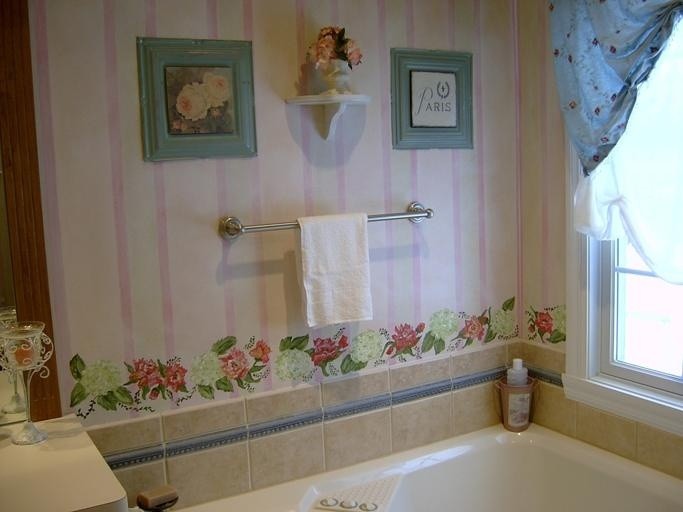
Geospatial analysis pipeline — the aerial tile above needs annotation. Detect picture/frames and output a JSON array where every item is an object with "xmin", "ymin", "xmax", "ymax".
[
  {"xmin": 388, "ymin": 44, "xmax": 474, "ymax": 150},
  {"xmin": 134, "ymin": 33, "xmax": 256, "ymax": 162}
]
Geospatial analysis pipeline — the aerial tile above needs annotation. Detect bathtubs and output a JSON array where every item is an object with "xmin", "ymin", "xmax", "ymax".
[{"xmin": 295, "ymin": 418, "xmax": 683, "ymax": 512}]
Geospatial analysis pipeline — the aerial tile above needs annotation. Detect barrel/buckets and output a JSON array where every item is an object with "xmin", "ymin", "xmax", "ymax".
[{"xmin": 494, "ymin": 378, "xmax": 539, "ymax": 431}]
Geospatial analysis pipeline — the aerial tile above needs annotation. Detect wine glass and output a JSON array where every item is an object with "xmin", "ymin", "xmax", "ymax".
[
  {"xmin": 1, "ymin": 320, "xmax": 55, "ymax": 446},
  {"xmin": 0, "ymin": 306, "xmax": 28, "ymax": 414}
]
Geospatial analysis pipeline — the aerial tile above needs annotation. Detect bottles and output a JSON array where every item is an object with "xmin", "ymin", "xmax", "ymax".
[{"xmin": 506, "ymin": 358, "xmax": 528, "ymax": 387}]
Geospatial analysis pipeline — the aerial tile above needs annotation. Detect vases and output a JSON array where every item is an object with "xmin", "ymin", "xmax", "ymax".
[{"xmin": 315, "ymin": 60, "xmax": 352, "ymax": 94}]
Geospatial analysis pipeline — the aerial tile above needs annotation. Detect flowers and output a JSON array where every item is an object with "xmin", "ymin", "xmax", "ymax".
[{"xmin": 306, "ymin": 23, "xmax": 363, "ymax": 69}]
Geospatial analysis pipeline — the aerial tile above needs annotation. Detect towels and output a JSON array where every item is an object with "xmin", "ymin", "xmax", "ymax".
[{"xmin": 295, "ymin": 212, "xmax": 373, "ymax": 329}]
